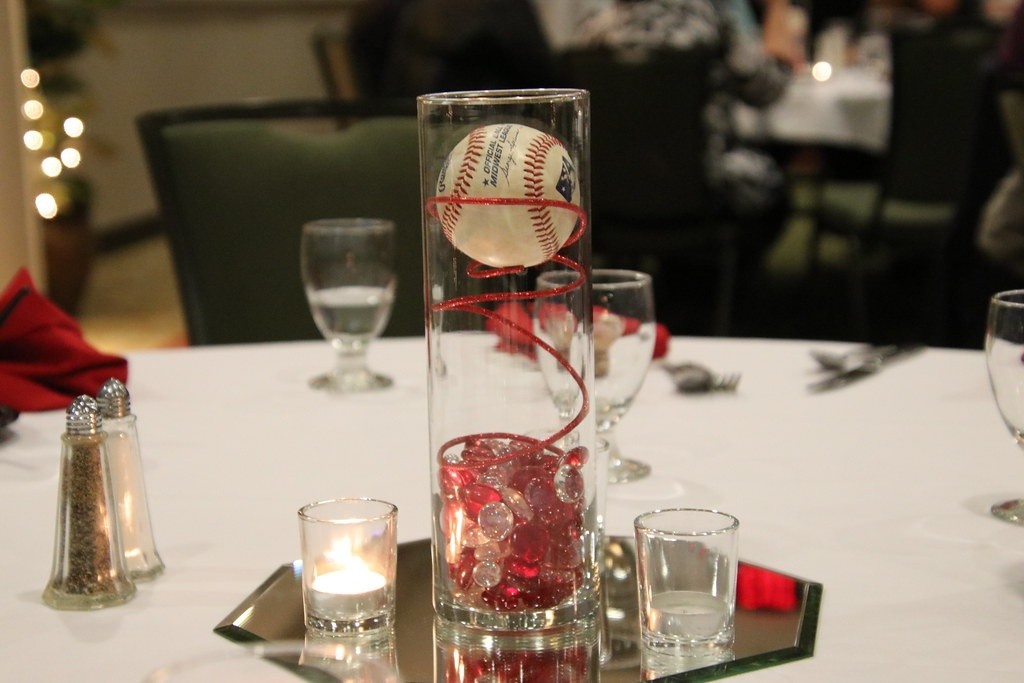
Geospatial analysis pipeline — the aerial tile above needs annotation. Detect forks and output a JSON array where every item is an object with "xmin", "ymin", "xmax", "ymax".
[
  {"xmin": 836, "ymin": 345, "xmax": 900, "ymax": 374},
  {"xmin": 707, "ymin": 373, "xmax": 741, "ymax": 389}
]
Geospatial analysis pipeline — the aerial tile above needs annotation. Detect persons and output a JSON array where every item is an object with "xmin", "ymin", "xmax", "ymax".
[{"xmin": 343, "ymin": 0, "xmax": 1024, "ymax": 287}]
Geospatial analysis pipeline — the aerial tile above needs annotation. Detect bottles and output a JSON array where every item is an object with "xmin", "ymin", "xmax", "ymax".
[{"xmin": 39, "ymin": 378, "xmax": 166, "ymax": 613}]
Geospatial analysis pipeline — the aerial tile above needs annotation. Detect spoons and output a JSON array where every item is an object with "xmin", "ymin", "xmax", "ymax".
[
  {"xmin": 809, "ymin": 351, "xmax": 846, "ymax": 369},
  {"xmin": 661, "ymin": 362, "xmax": 715, "ymax": 391}
]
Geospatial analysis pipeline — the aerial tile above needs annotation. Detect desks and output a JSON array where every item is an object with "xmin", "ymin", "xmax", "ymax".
[{"xmin": 0, "ymin": 331, "xmax": 1024, "ymax": 683}]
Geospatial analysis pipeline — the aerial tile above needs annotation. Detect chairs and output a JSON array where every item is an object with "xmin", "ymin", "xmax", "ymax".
[
  {"xmin": 325, "ymin": 0, "xmax": 1024, "ymax": 346},
  {"xmin": 133, "ymin": 94, "xmax": 484, "ymax": 346}
]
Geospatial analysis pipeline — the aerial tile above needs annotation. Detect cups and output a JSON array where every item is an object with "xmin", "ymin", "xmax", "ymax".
[
  {"xmin": 417, "ymin": 89, "xmax": 601, "ymax": 635},
  {"xmin": 297, "ymin": 497, "xmax": 397, "ymax": 641},
  {"xmin": 632, "ymin": 508, "xmax": 739, "ymax": 681}
]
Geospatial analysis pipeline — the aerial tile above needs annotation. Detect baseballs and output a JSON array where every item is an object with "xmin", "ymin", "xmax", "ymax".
[{"xmin": 432, "ymin": 120, "xmax": 579, "ymax": 268}]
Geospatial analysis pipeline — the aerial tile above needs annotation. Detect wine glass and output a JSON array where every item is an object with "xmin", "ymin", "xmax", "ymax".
[
  {"xmin": 985, "ymin": 289, "xmax": 1024, "ymax": 527},
  {"xmin": 297, "ymin": 218, "xmax": 398, "ymax": 395},
  {"xmin": 532, "ymin": 270, "xmax": 657, "ymax": 483}
]
